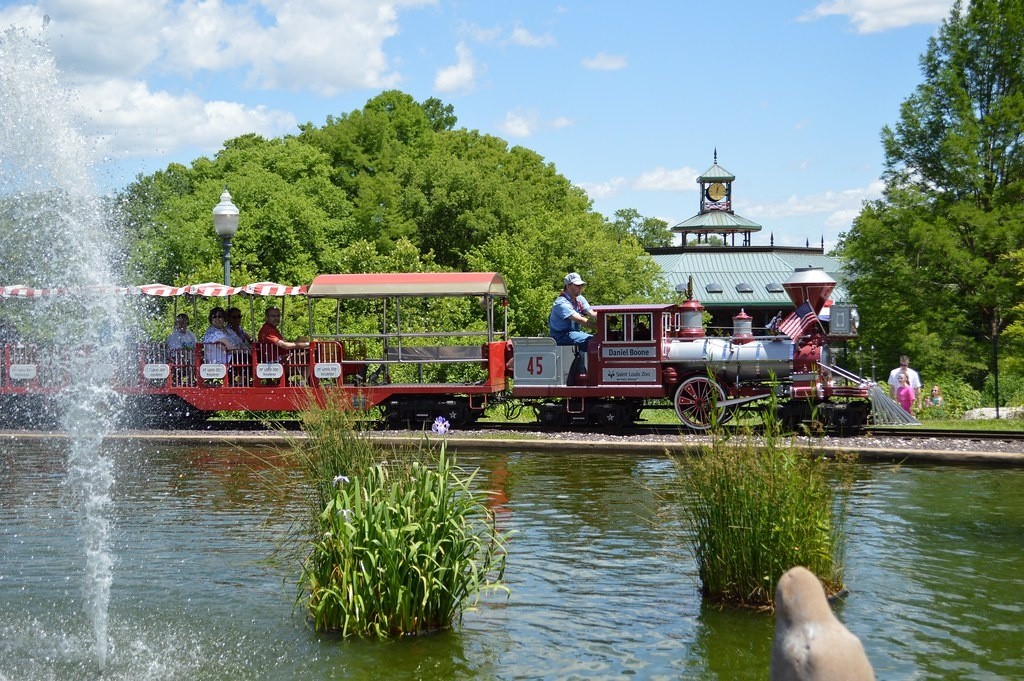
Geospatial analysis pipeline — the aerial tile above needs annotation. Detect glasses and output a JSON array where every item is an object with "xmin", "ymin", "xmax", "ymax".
[
  {"xmin": 901, "ymin": 364, "xmax": 907, "ymax": 367},
  {"xmin": 212, "ymin": 314, "xmax": 223, "ymax": 319},
  {"xmin": 267, "ymin": 314, "xmax": 281, "ymax": 318},
  {"xmin": 229, "ymin": 314, "xmax": 244, "ymax": 319}
]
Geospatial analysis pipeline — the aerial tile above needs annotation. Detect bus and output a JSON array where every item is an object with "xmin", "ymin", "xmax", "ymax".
[{"xmin": 1, "ymin": 267, "xmax": 868, "ymax": 430}]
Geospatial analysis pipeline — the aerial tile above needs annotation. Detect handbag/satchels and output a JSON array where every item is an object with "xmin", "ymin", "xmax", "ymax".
[{"xmin": 566, "ymin": 345, "xmax": 588, "ymax": 384}]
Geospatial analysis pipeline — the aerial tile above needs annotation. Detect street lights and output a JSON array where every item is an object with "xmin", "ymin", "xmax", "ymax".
[{"xmin": 212, "ymin": 188, "xmax": 239, "ymax": 288}]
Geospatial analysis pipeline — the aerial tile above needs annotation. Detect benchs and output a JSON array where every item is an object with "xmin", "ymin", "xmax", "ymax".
[
  {"xmin": 0, "ymin": 339, "xmax": 366, "ymax": 389},
  {"xmin": 511, "ymin": 337, "xmax": 588, "ymax": 384}
]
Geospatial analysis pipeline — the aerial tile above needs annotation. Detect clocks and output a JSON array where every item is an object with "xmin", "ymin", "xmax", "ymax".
[{"xmin": 706, "ymin": 182, "xmax": 727, "ymax": 201}]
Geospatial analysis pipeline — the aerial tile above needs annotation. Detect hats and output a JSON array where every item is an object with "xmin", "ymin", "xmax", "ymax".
[{"xmin": 564, "ymin": 273, "xmax": 586, "ymax": 286}]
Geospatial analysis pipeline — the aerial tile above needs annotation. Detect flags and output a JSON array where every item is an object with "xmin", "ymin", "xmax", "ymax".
[{"xmin": 777, "ymin": 302, "xmax": 819, "ymax": 343}]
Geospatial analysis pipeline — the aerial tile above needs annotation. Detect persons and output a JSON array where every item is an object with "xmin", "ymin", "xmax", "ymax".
[
  {"xmin": 549, "ymin": 272, "xmax": 618, "ymax": 353},
  {"xmin": 925, "ymin": 386, "xmax": 944, "ymax": 407},
  {"xmin": 896, "ymin": 373, "xmax": 917, "ymax": 416},
  {"xmin": 258, "ymin": 307, "xmax": 315, "ymax": 387},
  {"xmin": 887, "ymin": 356, "xmax": 923, "ymax": 411},
  {"xmin": 165, "ymin": 314, "xmax": 198, "ymax": 385},
  {"xmin": 203, "ymin": 307, "xmax": 260, "ymax": 386}
]
